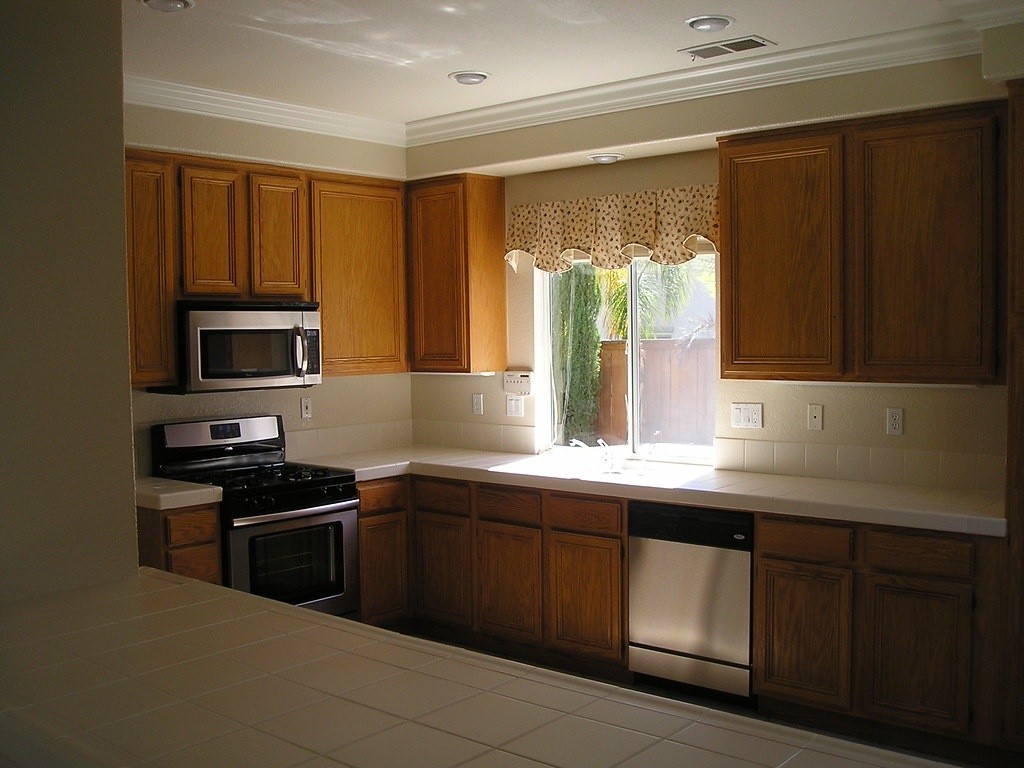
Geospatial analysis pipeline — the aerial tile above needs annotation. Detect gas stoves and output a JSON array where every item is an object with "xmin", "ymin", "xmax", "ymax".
[{"xmin": 149, "ymin": 415, "xmax": 355, "ymax": 498}]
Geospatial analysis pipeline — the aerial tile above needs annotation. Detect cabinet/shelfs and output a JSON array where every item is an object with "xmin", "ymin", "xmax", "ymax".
[
  {"xmin": 751, "ymin": 512, "xmax": 1007, "ymax": 750},
  {"xmin": 136, "ymin": 503, "xmax": 222, "ymax": 585},
  {"xmin": 716, "ymin": 98, "xmax": 1008, "ymax": 386},
  {"xmin": 355, "ymin": 474, "xmax": 627, "ymax": 673},
  {"xmin": 124, "ymin": 148, "xmax": 506, "ymax": 389}
]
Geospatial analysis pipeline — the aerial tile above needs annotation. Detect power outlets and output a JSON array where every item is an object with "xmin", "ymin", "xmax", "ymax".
[
  {"xmin": 301, "ymin": 397, "xmax": 312, "ymax": 419},
  {"xmin": 472, "ymin": 394, "xmax": 483, "ymax": 414},
  {"xmin": 887, "ymin": 408, "xmax": 903, "ymax": 435},
  {"xmin": 732, "ymin": 402, "xmax": 763, "ymax": 430}
]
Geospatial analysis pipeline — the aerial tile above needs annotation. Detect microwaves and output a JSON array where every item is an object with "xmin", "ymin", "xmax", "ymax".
[{"xmin": 146, "ymin": 299, "xmax": 322, "ymax": 396}]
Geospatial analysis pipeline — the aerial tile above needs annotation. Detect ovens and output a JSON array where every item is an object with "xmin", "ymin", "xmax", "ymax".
[{"xmin": 221, "ymin": 490, "xmax": 359, "ymax": 621}]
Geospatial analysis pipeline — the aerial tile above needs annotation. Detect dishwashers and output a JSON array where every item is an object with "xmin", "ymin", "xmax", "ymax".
[{"xmin": 626, "ymin": 501, "xmax": 753, "ymax": 697}]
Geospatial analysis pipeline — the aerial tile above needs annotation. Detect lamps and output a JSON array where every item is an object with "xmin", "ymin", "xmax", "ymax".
[
  {"xmin": 684, "ymin": 15, "xmax": 736, "ymax": 31},
  {"xmin": 589, "ymin": 154, "xmax": 623, "ymax": 164},
  {"xmin": 448, "ymin": 71, "xmax": 491, "ymax": 85},
  {"xmin": 140, "ymin": 0, "xmax": 196, "ymax": 12}
]
